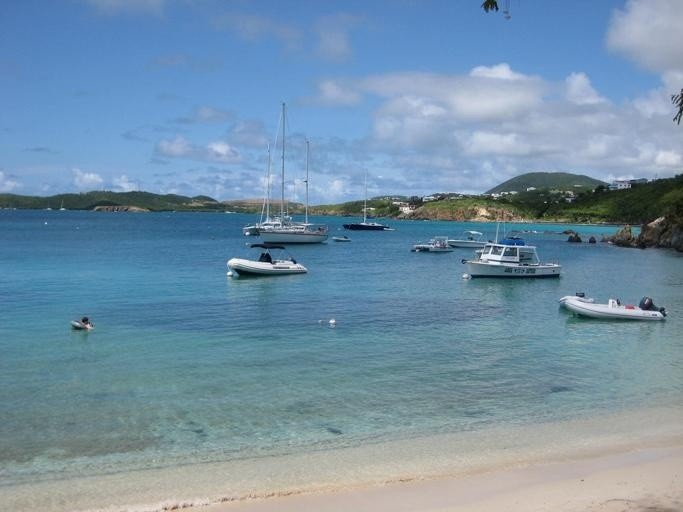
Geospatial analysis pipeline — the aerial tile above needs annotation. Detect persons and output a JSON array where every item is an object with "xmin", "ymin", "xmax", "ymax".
[{"xmin": 81, "ymin": 316, "xmax": 94, "ymax": 329}]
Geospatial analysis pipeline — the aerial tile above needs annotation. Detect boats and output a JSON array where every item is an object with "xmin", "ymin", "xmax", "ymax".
[
  {"xmin": 223, "ymin": 241, "xmax": 307, "ymax": 276},
  {"xmin": 330, "ymin": 234, "xmax": 351, "ymax": 243},
  {"xmin": 406, "ymin": 228, "xmax": 666, "ymax": 323}
]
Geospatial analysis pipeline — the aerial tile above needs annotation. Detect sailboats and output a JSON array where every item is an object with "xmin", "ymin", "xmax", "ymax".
[
  {"xmin": 341, "ymin": 171, "xmax": 388, "ymax": 230},
  {"xmin": 240, "ymin": 96, "xmax": 330, "ymax": 246}
]
